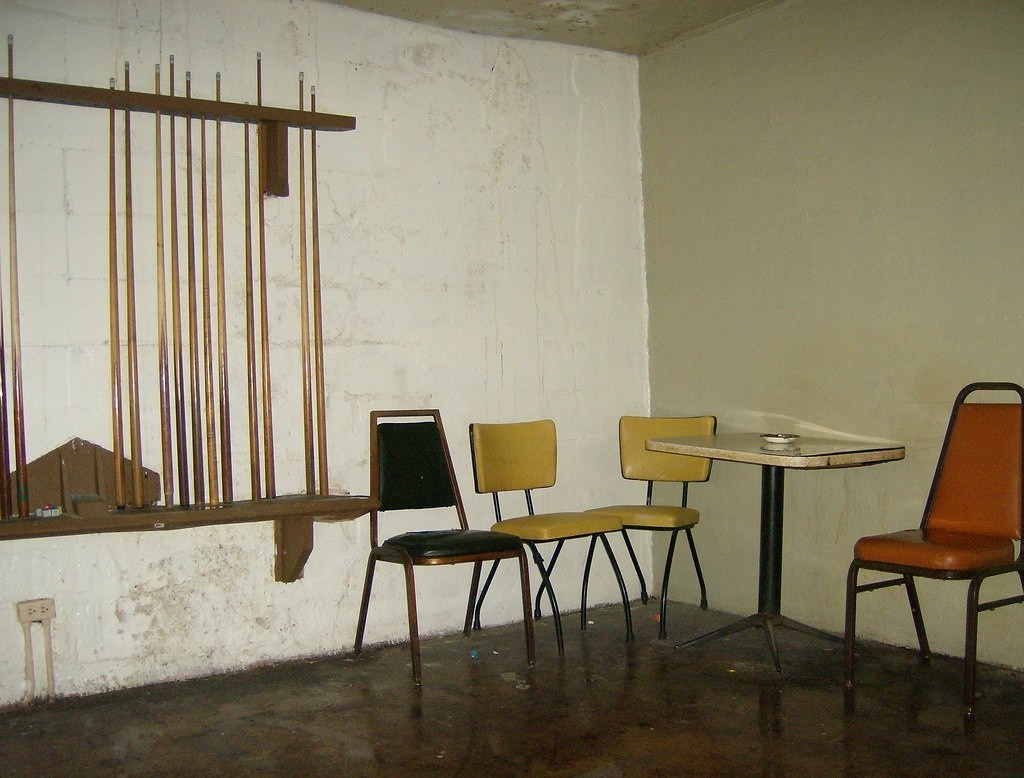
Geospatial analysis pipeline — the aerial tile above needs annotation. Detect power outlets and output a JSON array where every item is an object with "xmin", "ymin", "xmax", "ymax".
[{"xmin": 17, "ymin": 598, "xmax": 57, "ymax": 623}]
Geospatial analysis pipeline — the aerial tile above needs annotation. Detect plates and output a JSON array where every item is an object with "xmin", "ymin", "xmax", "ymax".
[{"xmin": 760, "ymin": 433, "xmax": 800, "ymax": 444}]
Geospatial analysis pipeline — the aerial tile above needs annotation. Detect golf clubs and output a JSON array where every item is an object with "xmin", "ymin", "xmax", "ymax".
[{"xmin": 1, "ymin": 35, "xmax": 328, "ymax": 519}]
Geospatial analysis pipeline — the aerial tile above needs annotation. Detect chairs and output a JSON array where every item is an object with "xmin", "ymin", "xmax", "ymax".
[
  {"xmin": 845, "ymin": 383, "xmax": 1024, "ymax": 719},
  {"xmin": 578, "ymin": 416, "xmax": 717, "ymax": 638},
  {"xmin": 354, "ymin": 409, "xmax": 535, "ymax": 686},
  {"xmin": 467, "ymin": 419, "xmax": 633, "ymax": 658}
]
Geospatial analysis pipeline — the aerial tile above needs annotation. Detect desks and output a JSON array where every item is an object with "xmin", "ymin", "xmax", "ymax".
[{"xmin": 645, "ymin": 433, "xmax": 904, "ymax": 673}]
